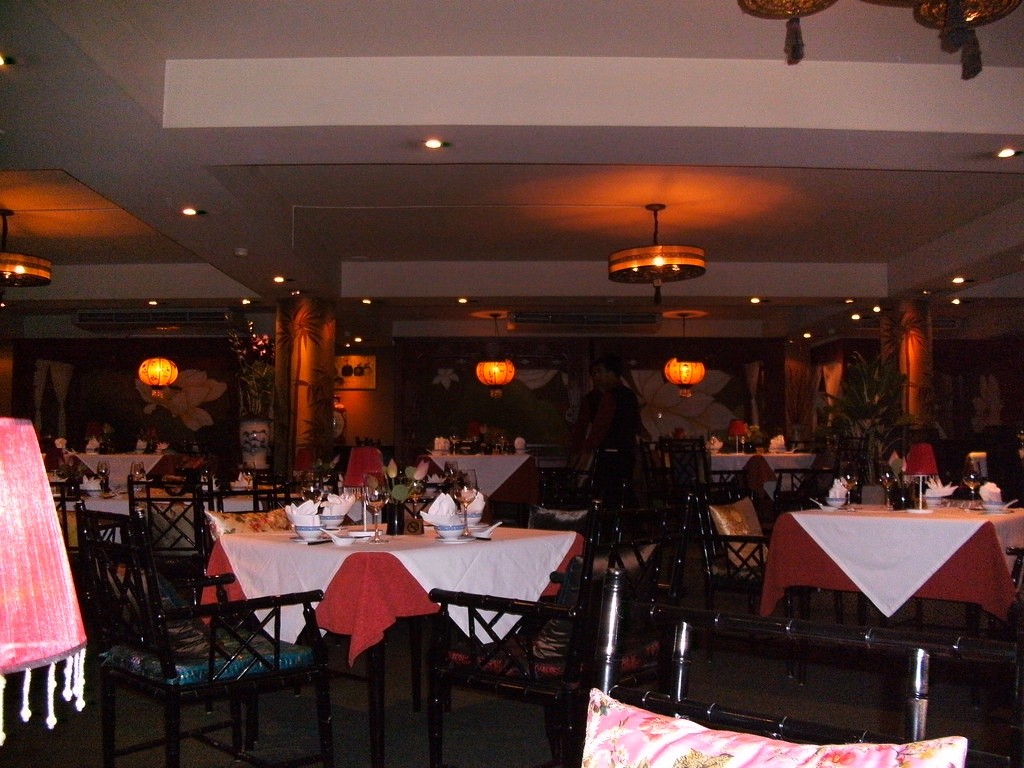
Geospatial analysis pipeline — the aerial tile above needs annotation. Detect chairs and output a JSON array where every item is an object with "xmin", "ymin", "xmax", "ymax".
[{"xmin": 47, "ymin": 428, "xmax": 1023, "ymax": 768}]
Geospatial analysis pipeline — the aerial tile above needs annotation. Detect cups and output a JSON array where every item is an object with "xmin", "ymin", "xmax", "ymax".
[{"xmin": 130, "ymin": 462, "xmax": 145, "ymax": 480}]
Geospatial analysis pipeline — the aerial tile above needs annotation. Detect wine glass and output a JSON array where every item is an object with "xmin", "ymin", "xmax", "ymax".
[
  {"xmin": 409, "ymin": 484, "xmax": 425, "ymax": 518},
  {"xmin": 362, "ymin": 473, "xmax": 390, "ymax": 544},
  {"xmin": 97, "ymin": 460, "xmax": 110, "ymax": 493},
  {"xmin": 963, "ymin": 457, "xmax": 984, "ymax": 500},
  {"xmin": 453, "ymin": 469, "xmax": 478, "ymax": 537}
]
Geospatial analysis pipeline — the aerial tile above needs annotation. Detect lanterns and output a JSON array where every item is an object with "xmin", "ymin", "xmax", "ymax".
[
  {"xmin": 664, "ymin": 357, "xmax": 705, "ymax": 397},
  {"xmin": 476, "ymin": 358, "xmax": 515, "ymax": 398},
  {"xmin": 138, "ymin": 357, "xmax": 178, "ymax": 398}
]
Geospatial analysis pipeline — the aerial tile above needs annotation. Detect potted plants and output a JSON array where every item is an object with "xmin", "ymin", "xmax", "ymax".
[
  {"xmin": 820, "ymin": 360, "xmax": 917, "ymax": 504},
  {"xmin": 230, "ymin": 306, "xmax": 274, "ymax": 470}
]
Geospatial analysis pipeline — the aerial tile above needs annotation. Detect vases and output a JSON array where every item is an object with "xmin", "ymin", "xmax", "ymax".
[{"xmin": 384, "ymin": 502, "xmax": 407, "ymax": 536}]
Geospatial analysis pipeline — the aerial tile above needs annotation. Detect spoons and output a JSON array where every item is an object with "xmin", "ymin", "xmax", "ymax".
[{"xmin": 472, "ymin": 521, "xmax": 503, "ymax": 537}]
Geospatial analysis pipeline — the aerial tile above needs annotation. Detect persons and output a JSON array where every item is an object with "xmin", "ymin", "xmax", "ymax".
[{"xmin": 569, "ymin": 353, "xmax": 653, "ymax": 543}]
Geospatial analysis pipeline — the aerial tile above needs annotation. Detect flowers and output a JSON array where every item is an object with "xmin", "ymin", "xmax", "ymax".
[{"xmin": 380, "ymin": 458, "xmax": 429, "ymax": 500}]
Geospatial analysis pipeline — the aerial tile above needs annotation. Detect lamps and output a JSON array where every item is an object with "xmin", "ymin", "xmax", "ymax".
[
  {"xmin": 0, "ymin": 415, "xmax": 89, "ymax": 741},
  {"xmin": 609, "ymin": 206, "xmax": 707, "ymax": 304},
  {"xmin": 474, "ymin": 313, "xmax": 514, "ymax": 398},
  {"xmin": 138, "ymin": 328, "xmax": 178, "ymax": 399},
  {"xmin": 666, "ymin": 316, "xmax": 708, "ymax": 399},
  {"xmin": 0, "ymin": 209, "xmax": 54, "ymax": 307},
  {"xmin": 728, "ymin": 419, "xmax": 748, "ymax": 453},
  {"xmin": 906, "ymin": 443, "xmax": 939, "ymax": 509}
]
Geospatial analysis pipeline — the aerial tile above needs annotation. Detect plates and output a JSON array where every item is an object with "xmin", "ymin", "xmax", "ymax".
[{"xmin": 332, "ymin": 538, "xmax": 356, "ymax": 547}]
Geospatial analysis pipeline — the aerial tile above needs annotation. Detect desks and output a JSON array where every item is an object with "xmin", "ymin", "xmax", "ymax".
[
  {"xmin": 663, "ymin": 449, "xmax": 816, "ymax": 493},
  {"xmin": 200, "ymin": 528, "xmax": 585, "ymax": 709},
  {"xmin": 58, "ymin": 492, "xmax": 262, "ymax": 589},
  {"xmin": 424, "ymin": 448, "xmax": 536, "ymax": 519},
  {"xmin": 763, "ymin": 500, "xmax": 1022, "ymax": 633}
]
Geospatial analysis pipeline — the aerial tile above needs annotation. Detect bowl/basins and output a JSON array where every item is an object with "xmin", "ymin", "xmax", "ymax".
[
  {"xmin": 825, "ymin": 497, "xmax": 847, "ymax": 508},
  {"xmin": 294, "ymin": 525, "xmax": 327, "ymax": 540},
  {"xmin": 434, "ymin": 523, "xmax": 466, "ymax": 539},
  {"xmin": 458, "ymin": 513, "xmax": 482, "ymax": 526},
  {"xmin": 318, "ymin": 514, "xmax": 344, "ymax": 529},
  {"xmin": 922, "ymin": 495, "xmax": 944, "ymax": 506},
  {"xmin": 980, "ymin": 501, "xmax": 1005, "ymax": 511}
]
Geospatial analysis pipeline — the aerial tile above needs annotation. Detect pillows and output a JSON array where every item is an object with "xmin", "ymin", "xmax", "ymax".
[
  {"xmin": 584, "ymin": 690, "xmax": 968, "ymax": 768},
  {"xmin": 707, "ymin": 497, "xmax": 768, "ymax": 569},
  {"xmin": 201, "ymin": 508, "xmax": 294, "ymax": 537}
]
